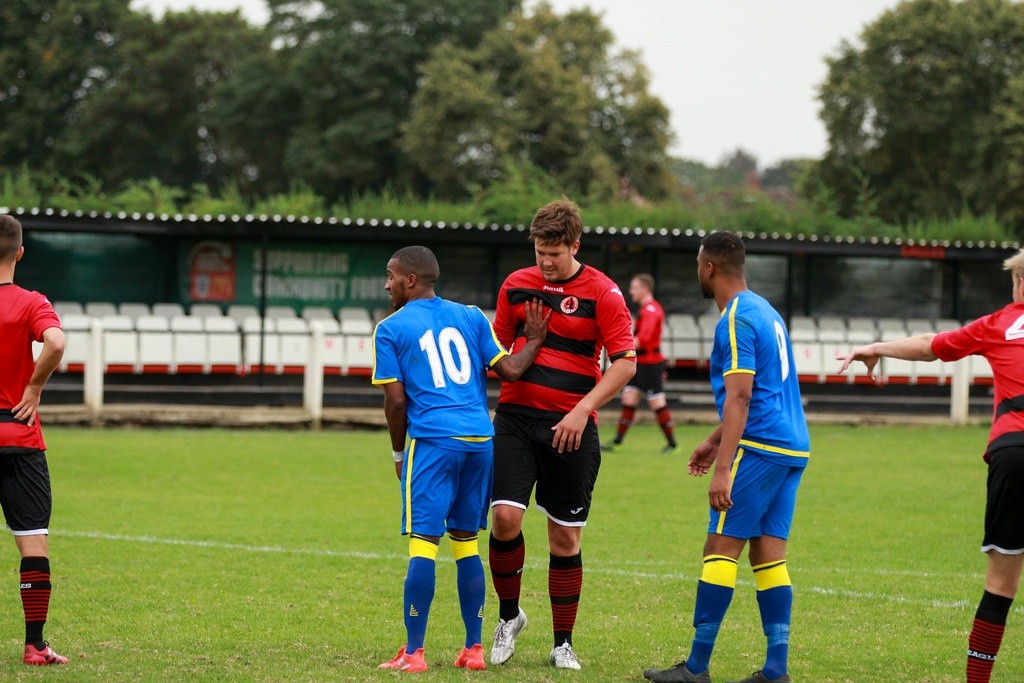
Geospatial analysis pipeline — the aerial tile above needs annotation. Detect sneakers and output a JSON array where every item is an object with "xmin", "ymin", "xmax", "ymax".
[
  {"xmin": 644, "ymin": 662, "xmax": 711, "ymax": 683},
  {"xmin": 727, "ymin": 672, "xmax": 791, "ymax": 683},
  {"xmin": 455, "ymin": 644, "xmax": 487, "ymax": 670},
  {"xmin": 377, "ymin": 646, "xmax": 427, "ymax": 672},
  {"xmin": 550, "ymin": 642, "xmax": 581, "ymax": 671},
  {"xmin": 24, "ymin": 644, "xmax": 68, "ymax": 665},
  {"xmin": 490, "ymin": 606, "xmax": 527, "ymax": 665}
]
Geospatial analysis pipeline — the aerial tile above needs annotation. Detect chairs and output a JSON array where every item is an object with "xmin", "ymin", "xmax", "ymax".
[{"xmin": 30, "ymin": 304, "xmax": 994, "ymax": 385}]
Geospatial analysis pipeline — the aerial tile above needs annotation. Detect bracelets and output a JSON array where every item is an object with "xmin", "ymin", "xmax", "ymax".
[{"xmin": 393, "ymin": 448, "xmax": 404, "ymax": 463}]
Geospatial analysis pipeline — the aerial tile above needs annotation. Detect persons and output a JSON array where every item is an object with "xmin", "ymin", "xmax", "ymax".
[
  {"xmin": 0, "ymin": 211, "xmax": 72, "ymax": 667},
  {"xmin": 599, "ymin": 271, "xmax": 680, "ymax": 458},
  {"xmin": 640, "ymin": 229, "xmax": 812, "ymax": 683},
  {"xmin": 481, "ymin": 191, "xmax": 638, "ymax": 672},
  {"xmin": 369, "ymin": 244, "xmax": 553, "ymax": 676},
  {"xmin": 834, "ymin": 244, "xmax": 1024, "ymax": 683}
]
